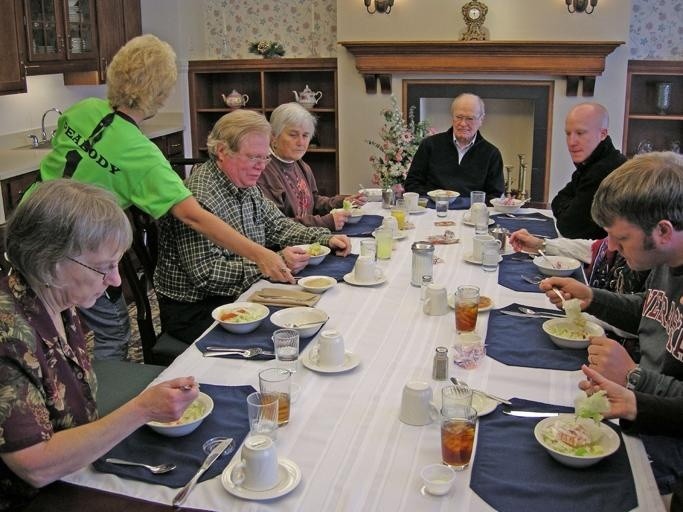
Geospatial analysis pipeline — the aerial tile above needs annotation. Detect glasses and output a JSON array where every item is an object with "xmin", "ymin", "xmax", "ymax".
[
  {"xmin": 66, "ymin": 256, "xmax": 114, "ymax": 281},
  {"xmin": 247, "ymin": 154, "xmax": 272, "ymax": 165}
]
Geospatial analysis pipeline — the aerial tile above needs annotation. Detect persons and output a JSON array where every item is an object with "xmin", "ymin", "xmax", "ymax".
[
  {"xmin": 577, "ymin": 364, "xmax": 683, "ymax": 512},
  {"xmin": 537, "ymin": 149, "xmax": 682, "ymax": 495},
  {"xmin": 257, "ymin": 102, "xmax": 371, "ymax": 253},
  {"xmin": 549, "ymin": 102, "xmax": 628, "ymax": 240},
  {"xmin": 511, "ymin": 226, "xmax": 652, "ymax": 363},
  {"xmin": 0, "ymin": 180, "xmax": 199, "ymax": 511},
  {"xmin": 152, "ymin": 109, "xmax": 354, "ymax": 344},
  {"xmin": 30, "ymin": 33, "xmax": 297, "ymax": 361},
  {"xmin": 403, "ymin": 91, "xmax": 505, "ymax": 208}
]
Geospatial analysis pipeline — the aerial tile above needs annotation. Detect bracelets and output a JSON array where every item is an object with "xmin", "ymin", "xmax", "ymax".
[{"xmin": 538, "ymin": 240, "xmax": 548, "ymax": 256}]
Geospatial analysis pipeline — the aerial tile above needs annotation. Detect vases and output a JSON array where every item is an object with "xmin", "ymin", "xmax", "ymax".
[{"xmin": 263, "ymin": 54, "xmax": 280, "ymax": 60}]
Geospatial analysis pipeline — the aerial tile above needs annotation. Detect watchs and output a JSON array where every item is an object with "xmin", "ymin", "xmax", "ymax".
[{"xmin": 623, "ymin": 363, "xmax": 648, "ymax": 394}]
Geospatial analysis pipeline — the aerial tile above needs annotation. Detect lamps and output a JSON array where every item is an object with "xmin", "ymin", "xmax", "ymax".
[
  {"xmin": 565, "ymin": 0, "xmax": 598, "ymax": 15},
  {"xmin": 364, "ymin": 0, "xmax": 394, "ymax": 15}
]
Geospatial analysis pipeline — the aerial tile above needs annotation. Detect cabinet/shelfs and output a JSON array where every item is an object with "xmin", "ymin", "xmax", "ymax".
[
  {"xmin": 621, "ymin": 58, "xmax": 683, "ymax": 162},
  {"xmin": 0, "ymin": 169, "xmax": 43, "ymax": 224},
  {"xmin": 21, "ymin": 1, "xmax": 102, "ymax": 77},
  {"xmin": 0, "ymin": 1, "xmax": 29, "ymax": 96},
  {"xmin": 186, "ymin": 56, "xmax": 339, "ymax": 198},
  {"xmin": 151, "ymin": 130, "xmax": 186, "ymax": 180},
  {"xmin": 63, "ymin": 0, "xmax": 144, "ymax": 88}
]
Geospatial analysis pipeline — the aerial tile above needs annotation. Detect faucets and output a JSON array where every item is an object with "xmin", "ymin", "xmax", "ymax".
[{"xmin": 40, "ymin": 106, "xmax": 64, "ymax": 142}]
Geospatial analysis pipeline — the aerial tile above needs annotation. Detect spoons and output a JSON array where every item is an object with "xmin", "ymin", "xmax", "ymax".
[
  {"xmin": 201, "ymin": 347, "xmax": 262, "ymax": 360},
  {"xmin": 103, "ymin": 455, "xmax": 178, "ymax": 476},
  {"xmin": 277, "ymin": 319, "xmax": 328, "ymax": 330},
  {"xmin": 449, "ymin": 376, "xmax": 513, "ymax": 406},
  {"xmin": 518, "ymin": 305, "xmax": 567, "ymax": 318}
]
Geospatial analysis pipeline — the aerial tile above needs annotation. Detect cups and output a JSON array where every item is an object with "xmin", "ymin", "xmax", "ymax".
[
  {"xmin": 374, "ymin": 228, "xmax": 394, "ymax": 260},
  {"xmin": 435, "ymin": 194, "xmax": 449, "ymax": 217},
  {"xmin": 471, "ymin": 235, "xmax": 494, "ymax": 261},
  {"xmin": 257, "ymin": 366, "xmax": 291, "ymax": 427},
  {"xmin": 418, "ymin": 198, "xmax": 428, "ymax": 210},
  {"xmin": 359, "ymin": 239, "xmax": 379, "ymax": 264},
  {"xmin": 419, "ymin": 486, "xmax": 455, "ymax": 512},
  {"xmin": 272, "ymin": 328, "xmax": 300, "ymax": 377},
  {"xmin": 307, "ymin": 330, "xmax": 346, "ymax": 369},
  {"xmin": 481, "ymin": 242, "xmax": 497, "ymax": 272},
  {"xmin": 244, "ymin": 390, "xmax": 279, "ymax": 443},
  {"xmin": 420, "ymin": 463, "xmax": 455, "ymax": 494},
  {"xmin": 453, "ymin": 291, "xmax": 479, "ymax": 334},
  {"xmin": 381, "ymin": 216, "xmax": 398, "ymax": 236},
  {"xmin": 474, "ymin": 209, "xmax": 488, "ymax": 235},
  {"xmin": 457, "ymin": 285, "xmax": 480, "ymax": 296},
  {"xmin": 230, "ymin": 434, "xmax": 282, "ymax": 492},
  {"xmin": 469, "ymin": 191, "xmax": 485, "ymax": 205},
  {"xmin": 421, "ymin": 285, "xmax": 449, "ymax": 317},
  {"xmin": 390, "ymin": 207, "xmax": 405, "ymax": 229},
  {"xmin": 395, "ymin": 199, "xmax": 409, "ymax": 223},
  {"xmin": 439, "ymin": 403, "xmax": 477, "ymax": 471},
  {"xmin": 398, "ymin": 379, "xmax": 440, "ymax": 426},
  {"xmin": 402, "ymin": 192, "xmax": 418, "ymax": 211},
  {"xmin": 488, "ymin": 223, "xmax": 512, "ymax": 254},
  {"xmin": 354, "ymin": 257, "xmax": 383, "ymax": 283}
]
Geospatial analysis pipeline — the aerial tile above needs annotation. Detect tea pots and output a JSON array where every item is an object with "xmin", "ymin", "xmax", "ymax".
[
  {"xmin": 292, "ymin": 84, "xmax": 322, "ymax": 109},
  {"xmin": 220, "ymin": 89, "xmax": 249, "ymax": 110}
]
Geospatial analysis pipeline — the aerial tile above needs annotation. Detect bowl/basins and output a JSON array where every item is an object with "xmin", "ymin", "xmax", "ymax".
[
  {"xmin": 531, "ymin": 255, "xmax": 582, "ymax": 277},
  {"xmin": 210, "ymin": 302, "xmax": 269, "ymax": 335},
  {"xmin": 358, "ymin": 188, "xmax": 382, "ymax": 202},
  {"xmin": 290, "ymin": 244, "xmax": 330, "ymax": 267},
  {"xmin": 489, "ymin": 197, "xmax": 525, "ymax": 212},
  {"xmin": 533, "ymin": 415, "xmax": 621, "ymax": 470},
  {"xmin": 540, "ymin": 318, "xmax": 604, "ymax": 350},
  {"xmin": 297, "ymin": 274, "xmax": 337, "ymax": 293},
  {"xmin": 426, "ymin": 189, "xmax": 460, "ymax": 205},
  {"xmin": 269, "ymin": 306, "xmax": 328, "ymax": 338},
  {"xmin": 143, "ymin": 391, "xmax": 213, "ymax": 438},
  {"xmin": 329, "ymin": 208, "xmax": 364, "ymax": 225}
]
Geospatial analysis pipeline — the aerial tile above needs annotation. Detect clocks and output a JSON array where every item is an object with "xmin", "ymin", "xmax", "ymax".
[{"xmin": 458, "ymin": 0, "xmax": 491, "ymax": 43}]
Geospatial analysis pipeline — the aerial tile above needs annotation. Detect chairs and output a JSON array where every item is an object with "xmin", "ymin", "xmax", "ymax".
[
  {"xmin": 119, "ymin": 203, "xmax": 189, "ymax": 365},
  {"xmin": 0, "ymin": 227, "xmax": 12, "ymax": 279},
  {"xmin": 89, "ymin": 358, "xmax": 167, "ymax": 417}
]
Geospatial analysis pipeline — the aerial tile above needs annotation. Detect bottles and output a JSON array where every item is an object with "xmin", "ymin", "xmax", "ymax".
[{"xmin": 432, "ymin": 346, "xmax": 448, "ymax": 381}]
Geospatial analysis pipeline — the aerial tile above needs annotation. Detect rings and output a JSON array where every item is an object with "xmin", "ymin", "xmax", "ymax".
[
  {"xmin": 279, "ymin": 266, "xmax": 294, "ymax": 276},
  {"xmin": 591, "ymin": 354, "xmax": 595, "ymax": 364}
]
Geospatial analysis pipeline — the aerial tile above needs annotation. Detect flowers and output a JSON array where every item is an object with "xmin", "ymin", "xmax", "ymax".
[
  {"xmin": 365, "ymin": 94, "xmax": 434, "ymax": 197},
  {"xmin": 248, "ymin": 40, "xmax": 286, "ymax": 56}
]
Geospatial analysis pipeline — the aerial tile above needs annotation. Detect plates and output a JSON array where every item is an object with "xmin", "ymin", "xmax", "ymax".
[
  {"xmin": 371, "ymin": 229, "xmax": 408, "ymax": 240},
  {"xmin": 408, "ymin": 207, "xmax": 427, "ymax": 215},
  {"xmin": 220, "ymin": 455, "xmax": 301, "ymax": 502},
  {"xmin": 342, "ymin": 271, "xmax": 388, "ymax": 287},
  {"xmin": 441, "ymin": 391, "xmax": 498, "ymax": 417},
  {"xmin": 301, "ymin": 348, "xmax": 360, "ymax": 375},
  {"xmin": 464, "ymin": 252, "xmax": 503, "ymax": 264},
  {"xmin": 200, "ymin": 435, "xmax": 236, "ymax": 458},
  {"xmin": 463, "ymin": 218, "xmax": 495, "ymax": 227},
  {"xmin": 448, "ymin": 294, "xmax": 494, "ymax": 312}
]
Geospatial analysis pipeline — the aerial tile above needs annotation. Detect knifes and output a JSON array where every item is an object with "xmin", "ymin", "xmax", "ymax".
[
  {"xmin": 499, "ymin": 309, "xmax": 564, "ymax": 319},
  {"xmin": 501, "ymin": 409, "xmax": 575, "ymax": 418},
  {"xmin": 171, "ymin": 436, "xmax": 234, "ymax": 508}
]
